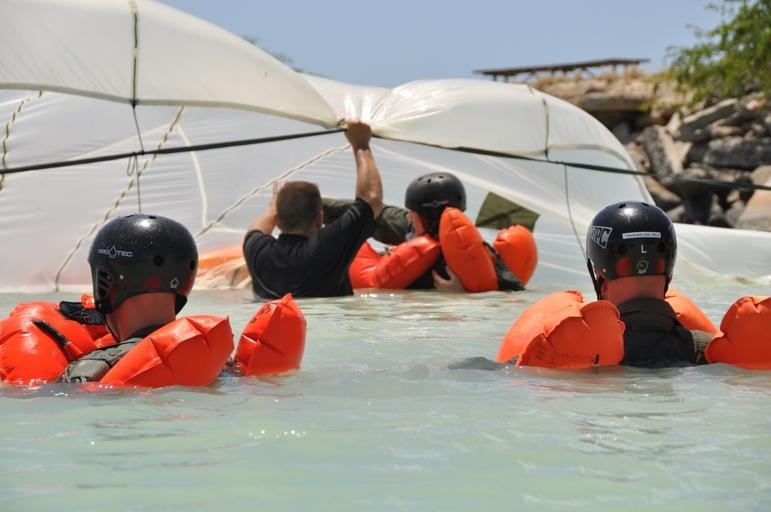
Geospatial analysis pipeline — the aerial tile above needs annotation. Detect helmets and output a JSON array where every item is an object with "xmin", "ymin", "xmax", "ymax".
[
  {"xmin": 405, "ymin": 173, "xmax": 467, "ymax": 231},
  {"xmin": 586, "ymin": 201, "xmax": 677, "ymax": 282},
  {"xmin": 88, "ymin": 213, "xmax": 198, "ymax": 316}
]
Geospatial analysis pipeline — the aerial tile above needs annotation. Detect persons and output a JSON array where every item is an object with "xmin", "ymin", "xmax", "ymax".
[
  {"xmin": 494, "ymin": 200, "xmax": 771, "ymax": 373},
  {"xmin": 322, "ymin": 172, "xmax": 525, "ymax": 293},
  {"xmin": 0, "ymin": 213, "xmax": 308, "ymax": 393},
  {"xmin": 244, "ymin": 121, "xmax": 383, "ymax": 301}
]
